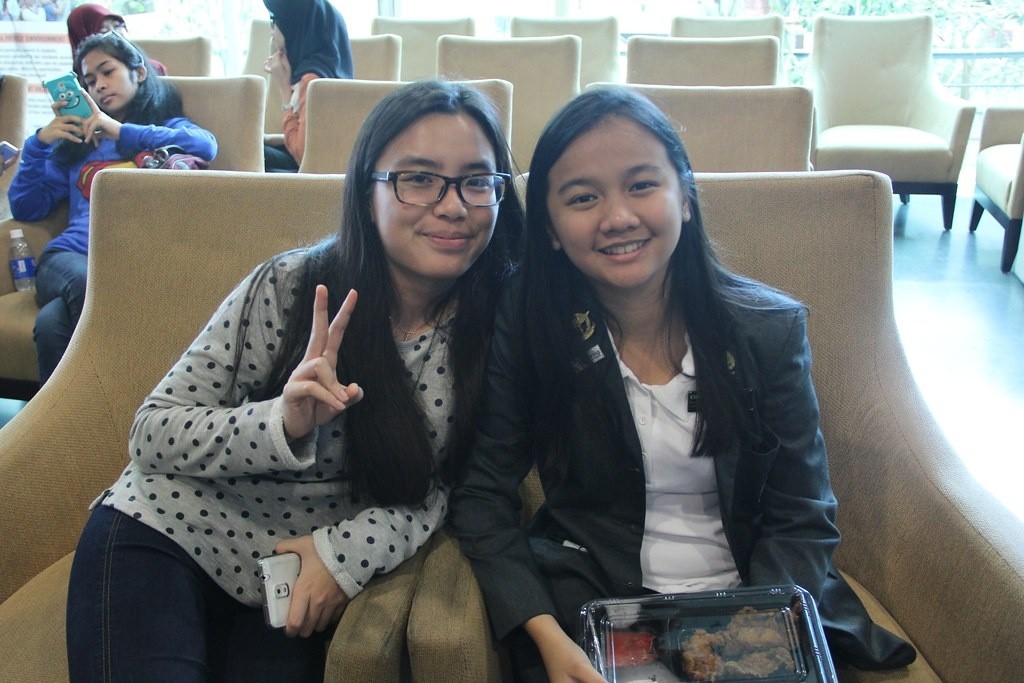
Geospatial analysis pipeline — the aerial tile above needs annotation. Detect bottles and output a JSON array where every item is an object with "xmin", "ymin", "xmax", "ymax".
[{"xmin": 10, "ymin": 229, "xmax": 37, "ymax": 293}]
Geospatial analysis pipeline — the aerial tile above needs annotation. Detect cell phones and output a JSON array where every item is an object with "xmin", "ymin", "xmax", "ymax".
[{"xmin": 46, "ymin": 73, "xmax": 94, "ymax": 138}]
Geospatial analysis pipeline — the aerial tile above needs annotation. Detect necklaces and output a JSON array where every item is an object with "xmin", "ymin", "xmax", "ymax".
[{"xmin": 387, "ymin": 309, "xmax": 431, "ymax": 341}]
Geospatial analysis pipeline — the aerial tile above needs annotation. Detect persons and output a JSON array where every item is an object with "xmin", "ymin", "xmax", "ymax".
[
  {"xmin": 442, "ymin": 88, "xmax": 918, "ymax": 683},
  {"xmin": 68, "ymin": 81, "xmax": 524, "ymax": 683},
  {"xmin": 8, "ymin": 32, "xmax": 218, "ymax": 389},
  {"xmin": 67, "ymin": 4, "xmax": 167, "ymax": 80},
  {"xmin": 265, "ymin": 0, "xmax": 353, "ymax": 170}
]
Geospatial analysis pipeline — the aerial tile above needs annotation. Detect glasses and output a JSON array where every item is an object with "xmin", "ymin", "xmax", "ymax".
[
  {"xmin": 114, "ymin": 24, "xmax": 128, "ymax": 35},
  {"xmin": 370, "ymin": 169, "xmax": 513, "ymax": 210},
  {"xmin": 270, "ymin": 15, "xmax": 277, "ymax": 28}
]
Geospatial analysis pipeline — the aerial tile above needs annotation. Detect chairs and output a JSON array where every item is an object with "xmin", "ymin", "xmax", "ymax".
[{"xmin": 1, "ymin": 17, "xmax": 1024, "ymax": 682}]
